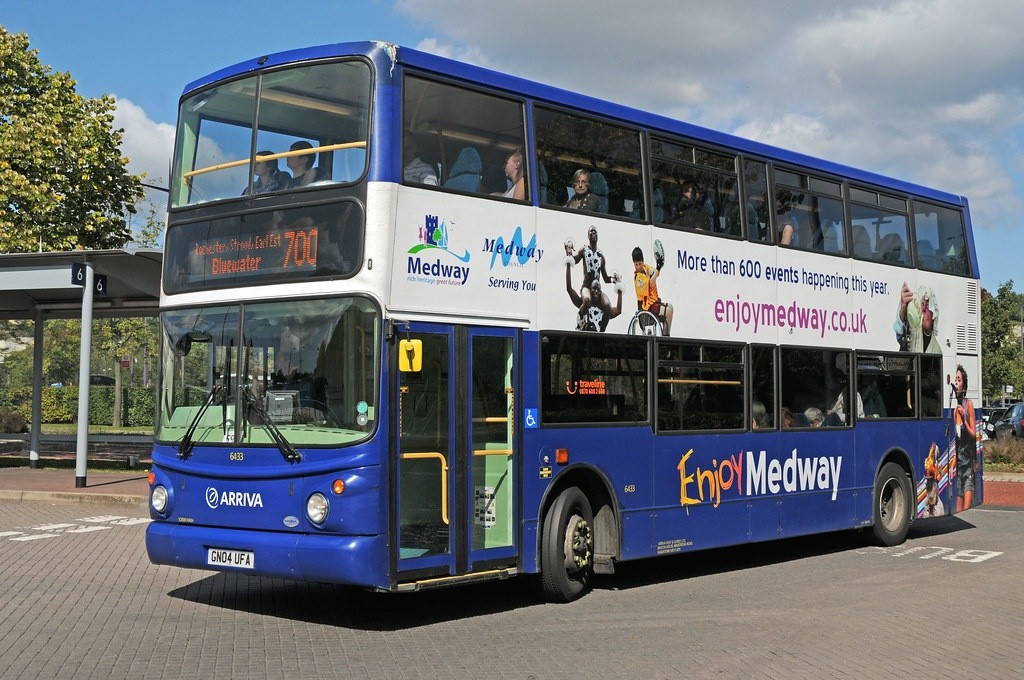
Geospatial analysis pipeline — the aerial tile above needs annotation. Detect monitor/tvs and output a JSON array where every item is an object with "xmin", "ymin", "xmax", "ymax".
[{"xmin": 265, "ymin": 390, "xmax": 303, "ymax": 423}]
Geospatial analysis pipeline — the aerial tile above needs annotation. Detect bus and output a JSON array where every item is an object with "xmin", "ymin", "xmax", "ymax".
[{"xmin": 136, "ymin": 42, "xmax": 982, "ymax": 602}]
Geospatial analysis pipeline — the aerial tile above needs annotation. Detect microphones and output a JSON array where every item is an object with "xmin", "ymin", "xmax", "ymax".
[{"xmin": 951, "ymin": 383, "xmax": 957, "ymax": 390}]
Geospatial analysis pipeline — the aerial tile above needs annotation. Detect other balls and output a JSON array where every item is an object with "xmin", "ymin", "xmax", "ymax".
[{"xmin": 652, "ymin": 239, "xmax": 664, "ymax": 266}]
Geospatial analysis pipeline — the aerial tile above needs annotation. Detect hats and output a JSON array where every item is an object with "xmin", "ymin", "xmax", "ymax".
[{"xmin": 913, "ymin": 285, "xmax": 940, "ymax": 336}]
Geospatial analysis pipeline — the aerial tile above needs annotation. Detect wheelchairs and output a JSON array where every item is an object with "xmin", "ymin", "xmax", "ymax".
[{"xmin": 627, "ymin": 297, "xmax": 665, "ymax": 336}]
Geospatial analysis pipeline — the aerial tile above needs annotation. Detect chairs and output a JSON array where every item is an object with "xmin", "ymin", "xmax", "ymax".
[
  {"xmin": 875, "ymin": 233, "xmax": 907, "ymax": 265},
  {"xmin": 790, "ymin": 412, "xmax": 810, "ymax": 428},
  {"xmin": 841, "ymin": 225, "xmax": 872, "ymax": 259},
  {"xmin": 916, "ymin": 240, "xmax": 936, "ymax": 272},
  {"xmin": 670, "ymin": 195, "xmax": 714, "ymax": 233},
  {"xmin": 936, "ymin": 255, "xmax": 968, "ymax": 276},
  {"xmin": 824, "ymin": 411, "xmax": 842, "ymax": 426},
  {"xmin": 443, "ymin": 147, "xmax": 484, "ymax": 193},
  {"xmin": 628, "ymin": 187, "xmax": 664, "ymax": 225},
  {"xmin": 788, "ymin": 216, "xmax": 800, "ymax": 246},
  {"xmin": 807, "ymin": 218, "xmax": 838, "ymax": 252},
  {"xmin": 729, "ymin": 203, "xmax": 758, "ymax": 240},
  {"xmin": 586, "ymin": 172, "xmax": 610, "ymax": 215},
  {"xmin": 539, "ymin": 159, "xmax": 549, "ymax": 203}
]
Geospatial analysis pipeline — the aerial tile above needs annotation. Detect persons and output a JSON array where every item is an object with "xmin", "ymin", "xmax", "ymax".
[
  {"xmin": 772, "ymin": 407, "xmax": 795, "ymax": 427},
  {"xmin": 684, "ymin": 370, "xmax": 719, "ymax": 410},
  {"xmin": 285, "ymin": 141, "xmax": 321, "ymax": 189},
  {"xmin": 778, "ymin": 217, "xmax": 794, "ymax": 245},
  {"xmin": 675, "ymin": 182, "xmax": 701, "ymax": 212},
  {"xmin": 241, "ymin": 151, "xmax": 284, "ymax": 196},
  {"xmin": 925, "ymin": 440, "xmax": 941, "ymax": 515},
  {"xmin": 752, "ymin": 401, "xmax": 765, "ymax": 429},
  {"xmin": 268, "ymin": 350, "xmax": 316, "ymax": 407},
  {"xmin": 632, "ymin": 247, "xmax": 673, "ymax": 336},
  {"xmin": 403, "ymin": 130, "xmax": 438, "ymax": 186},
  {"xmin": 953, "ymin": 365, "xmax": 976, "ymax": 512},
  {"xmin": 827, "ymin": 385, "xmax": 865, "ymax": 425},
  {"xmin": 564, "ymin": 228, "xmax": 623, "ymax": 333},
  {"xmin": 895, "ymin": 283, "xmax": 942, "ymax": 354},
  {"xmin": 567, "ymin": 169, "xmax": 599, "ymax": 212},
  {"xmin": 804, "ymin": 407, "xmax": 830, "ymax": 427},
  {"xmin": 501, "ymin": 149, "xmax": 525, "ymax": 200}
]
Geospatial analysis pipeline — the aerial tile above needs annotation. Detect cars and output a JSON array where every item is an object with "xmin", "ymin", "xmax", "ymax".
[{"xmin": 982, "ymin": 399, "xmax": 1024, "ymax": 441}]
